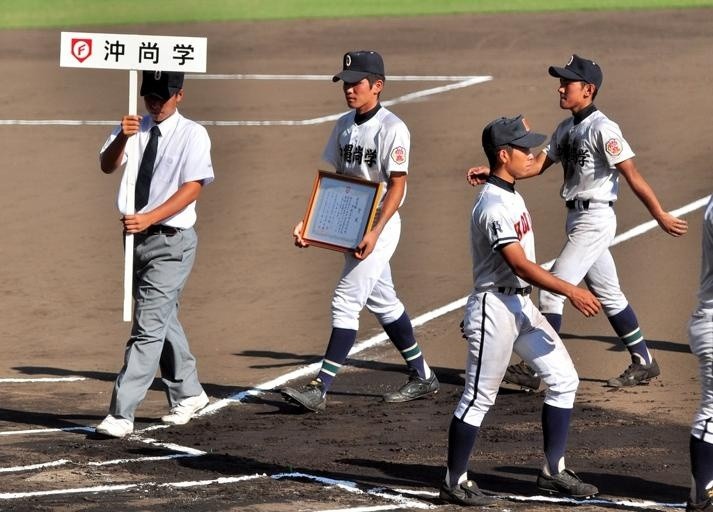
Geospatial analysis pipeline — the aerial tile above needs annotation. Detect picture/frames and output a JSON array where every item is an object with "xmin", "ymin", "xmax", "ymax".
[{"xmin": 299, "ymin": 168, "xmax": 385, "ymax": 256}]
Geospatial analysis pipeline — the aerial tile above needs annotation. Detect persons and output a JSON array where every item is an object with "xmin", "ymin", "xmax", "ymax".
[
  {"xmin": 683, "ymin": 182, "xmax": 713, "ymax": 511},
  {"xmin": 438, "ymin": 111, "xmax": 600, "ymax": 507},
  {"xmin": 94, "ymin": 72, "xmax": 211, "ymax": 440},
  {"xmin": 501, "ymin": 54, "xmax": 691, "ymax": 400},
  {"xmin": 280, "ymin": 49, "xmax": 440, "ymax": 413}
]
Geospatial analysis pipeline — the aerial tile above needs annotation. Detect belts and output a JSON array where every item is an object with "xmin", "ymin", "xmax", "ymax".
[
  {"xmin": 497, "ymin": 284, "xmax": 532, "ymax": 296},
  {"xmin": 564, "ymin": 198, "xmax": 613, "ymax": 208},
  {"xmin": 140, "ymin": 224, "xmax": 179, "ymax": 236}
]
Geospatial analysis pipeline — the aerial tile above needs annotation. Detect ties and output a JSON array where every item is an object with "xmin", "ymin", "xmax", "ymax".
[{"xmin": 134, "ymin": 125, "xmax": 161, "ymax": 211}]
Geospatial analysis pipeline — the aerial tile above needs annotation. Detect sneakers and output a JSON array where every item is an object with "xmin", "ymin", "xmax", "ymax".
[
  {"xmin": 161, "ymin": 391, "xmax": 209, "ymax": 425},
  {"xmin": 536, "ymin": 468, "xmax": 598, "ymax": 497},
  {"xmin": 96, "ymin": 414, "xmax": 134, "ymax": 438},
  {"xmin": 607, "ymin": 358, "xmax": 660, "ymax": 387},
  {"xmin": 385, "ymin": 371, "xmax": 439, "ymax": 402},
  {"xmin": 502, "ymin": 361, "xmax": 540, "ymax": 392},
  {"xmin": 440, "ymin": 478, "xmax": 498, "ymax": 506},
  {"xmin": 280, "ymin": 379, "xmax": 326, "ymax": 412}
]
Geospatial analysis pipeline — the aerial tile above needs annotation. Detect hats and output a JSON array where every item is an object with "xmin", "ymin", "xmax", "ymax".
[
  {"xmin": 140, "ymin": 71, "xmax": 183, "ymax": 96},
  {"xmin": 481, "ymin": 115, "xmax": 546, "ymax": 149},
  {"xmin": 548, "ymin": 54, "xmax": 603, "ymax": 90},
  {"xmin": 333, "ymin": 50, "xmax": 384, "ymax": 83}
]
